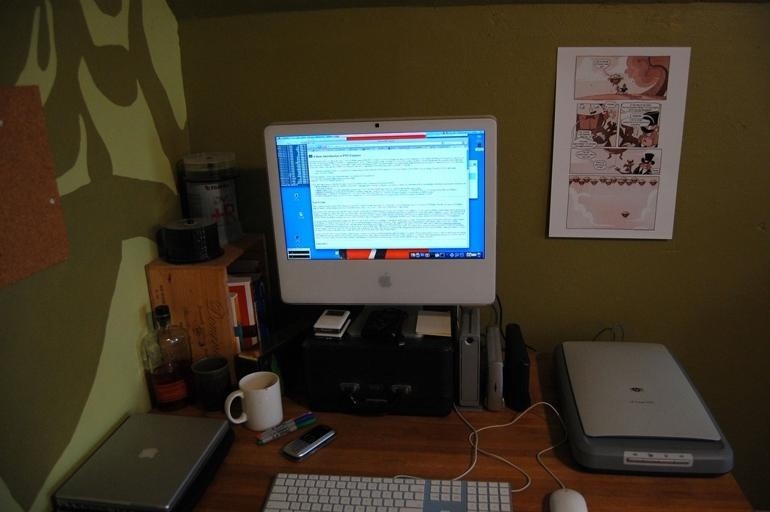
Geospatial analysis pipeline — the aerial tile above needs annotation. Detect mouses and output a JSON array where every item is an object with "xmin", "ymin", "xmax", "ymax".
[{"xmin": 549, "ymin": 487, "xmax": 588, "ymax": 512}]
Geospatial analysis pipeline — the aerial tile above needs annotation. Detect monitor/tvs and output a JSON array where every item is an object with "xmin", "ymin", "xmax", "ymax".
[{"xmin": 264, "ymin": 114, "xmax": 498, "ymax": 339}]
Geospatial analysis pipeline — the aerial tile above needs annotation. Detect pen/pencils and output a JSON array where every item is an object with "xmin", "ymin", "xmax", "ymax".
[{"xmin": 255, "ymin": 411, "xmax": 317, "ymax": 446}]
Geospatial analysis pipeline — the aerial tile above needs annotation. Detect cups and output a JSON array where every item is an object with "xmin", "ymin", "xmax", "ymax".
[{"xmin": 192, "ymin": 355, "xmax": 283, "ymax": 431}]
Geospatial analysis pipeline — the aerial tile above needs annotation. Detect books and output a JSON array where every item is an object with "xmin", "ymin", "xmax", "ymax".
[{"xmin": 228, "ymin": 258, "xmax": 270, "ymax": 361}]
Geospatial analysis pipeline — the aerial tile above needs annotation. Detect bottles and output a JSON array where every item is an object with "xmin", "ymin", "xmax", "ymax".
[{"xmin": 140, "ymin": 304, "xmax": 194, "ymax": 414}]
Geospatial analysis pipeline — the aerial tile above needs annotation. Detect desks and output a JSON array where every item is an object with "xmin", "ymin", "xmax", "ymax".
[{"xmin": 147, "ymin": 349, "xmax": 757, "ymax": 510}]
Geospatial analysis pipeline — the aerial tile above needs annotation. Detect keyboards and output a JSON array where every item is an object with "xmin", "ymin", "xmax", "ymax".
[{"xmin": 260, "ymin": 472, "xmax": 514, "ymax": 512}]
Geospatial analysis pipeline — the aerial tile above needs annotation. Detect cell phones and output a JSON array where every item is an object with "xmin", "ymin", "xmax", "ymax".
[{"xmin": 282, "ymin": 424, "xmax": 337, "ymax": 463}]
